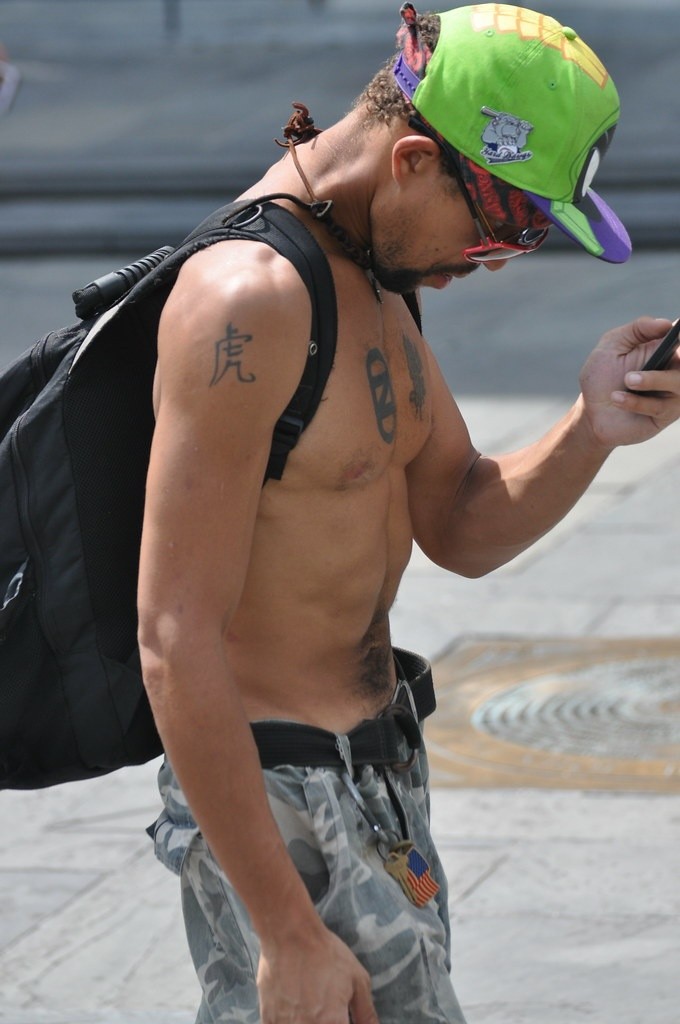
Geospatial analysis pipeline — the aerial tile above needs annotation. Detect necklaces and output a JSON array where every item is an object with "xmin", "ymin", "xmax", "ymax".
[{"xmin": 274, "ymin": 104, "xmax": 385, "ymax": 302}]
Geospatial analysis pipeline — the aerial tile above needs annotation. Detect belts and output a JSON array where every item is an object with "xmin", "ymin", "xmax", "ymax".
[{"xmin": 244, "ymin": 648, "xmax": 438, "ymax": 768}]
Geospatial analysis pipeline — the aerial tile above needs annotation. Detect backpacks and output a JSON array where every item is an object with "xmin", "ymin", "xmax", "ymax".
[{"xmin": 0, "ymin": 202, "xmax": 338, "ymax": 789}]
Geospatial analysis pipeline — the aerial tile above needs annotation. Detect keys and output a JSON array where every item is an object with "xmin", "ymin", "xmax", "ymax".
[{"xmin": 382, "ymin": 851, "xmax": 422, "ymax": 907}]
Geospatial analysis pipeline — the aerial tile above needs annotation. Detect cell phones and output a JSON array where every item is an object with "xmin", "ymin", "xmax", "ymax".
[{"xmin": 625, "ymin": 317, "xmax": 680, "ymax": 394}]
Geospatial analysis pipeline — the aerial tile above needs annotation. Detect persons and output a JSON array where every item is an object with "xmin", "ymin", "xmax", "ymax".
[{"xmin": 134, "ymin": 2, "xmax": 680, "ymax": 1022}]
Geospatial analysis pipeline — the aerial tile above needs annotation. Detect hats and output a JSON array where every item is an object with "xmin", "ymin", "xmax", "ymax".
[{"xmin": 391, "ymin": 3, "xmax": 632, "ymax": 264}]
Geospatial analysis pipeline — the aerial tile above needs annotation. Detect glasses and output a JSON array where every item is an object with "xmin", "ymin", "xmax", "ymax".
[{"xmin": 409, "ymin": 116, "xmax": 549, "ymax": 264}]
